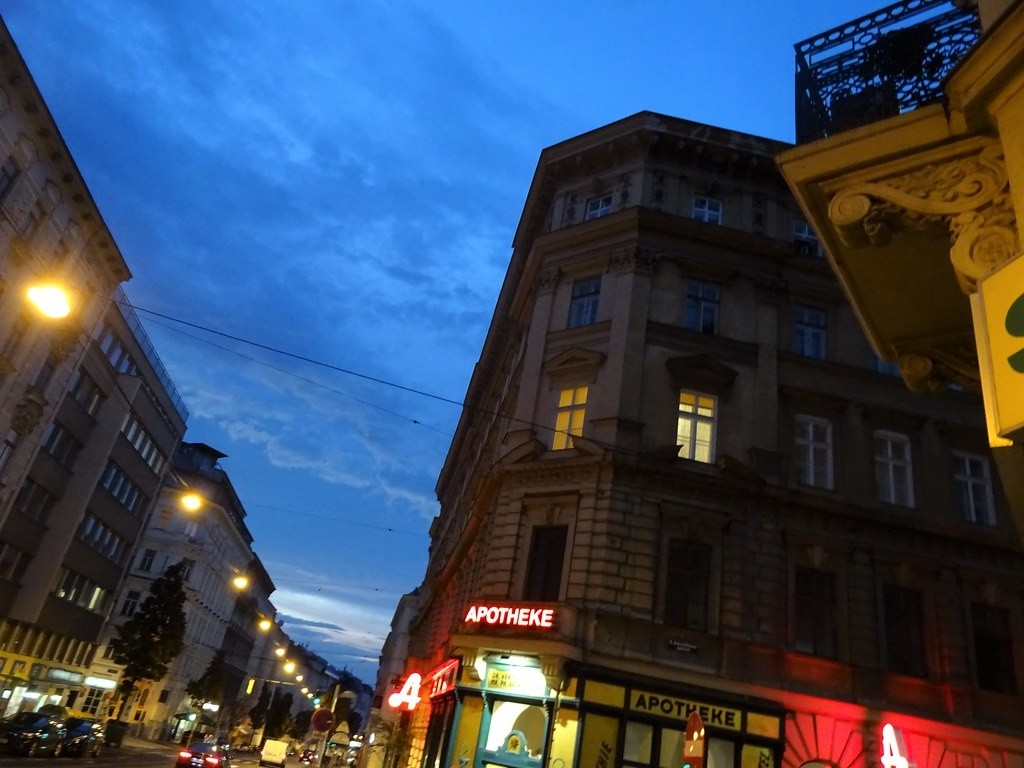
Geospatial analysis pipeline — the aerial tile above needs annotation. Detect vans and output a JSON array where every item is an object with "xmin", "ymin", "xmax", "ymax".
[{"xmin": 259, "ymin": 739, "xmax": 289, "ymax": 768}]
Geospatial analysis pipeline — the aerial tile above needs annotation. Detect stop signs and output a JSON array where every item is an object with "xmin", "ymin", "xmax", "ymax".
[{"xmin": 683, "ymin": 713, "xmax": 704, "ymax": 768}]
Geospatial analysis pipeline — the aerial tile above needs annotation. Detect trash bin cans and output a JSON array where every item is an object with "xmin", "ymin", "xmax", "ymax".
[{"xmin": 105, "ymin": 719, "xmax": 129, "ymax": 748}]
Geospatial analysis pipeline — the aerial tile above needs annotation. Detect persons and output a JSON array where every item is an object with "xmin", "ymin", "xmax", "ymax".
[{"xmin": 350, "ymin": 757, "xmax": 358, "ymax": 768}]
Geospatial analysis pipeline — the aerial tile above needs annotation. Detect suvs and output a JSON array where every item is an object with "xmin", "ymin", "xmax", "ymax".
[{"xmin": 298, "ymin": 749, "xmax": 314, "ymax": 764}]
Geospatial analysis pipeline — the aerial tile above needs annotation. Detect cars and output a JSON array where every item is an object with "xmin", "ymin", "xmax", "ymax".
[
  {"xmin": 175, "ymin": 743, "xmax": 234, "ymax": 768},
  {"xmin": 0, "ymin": 711, "xmax": 68, "ymax": 758},
  {"xmin": 38, "ymin": 704, "xmax": 75, "ymax": 721},
  {"xmin": 61, "ymin": 718, "xmax": 106, "ymax": 758}
]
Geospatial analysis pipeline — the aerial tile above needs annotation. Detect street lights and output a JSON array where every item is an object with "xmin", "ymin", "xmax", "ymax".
[{"xmin": 317, "ymin": 685, "xmax": 357, "ymax": 768}]
